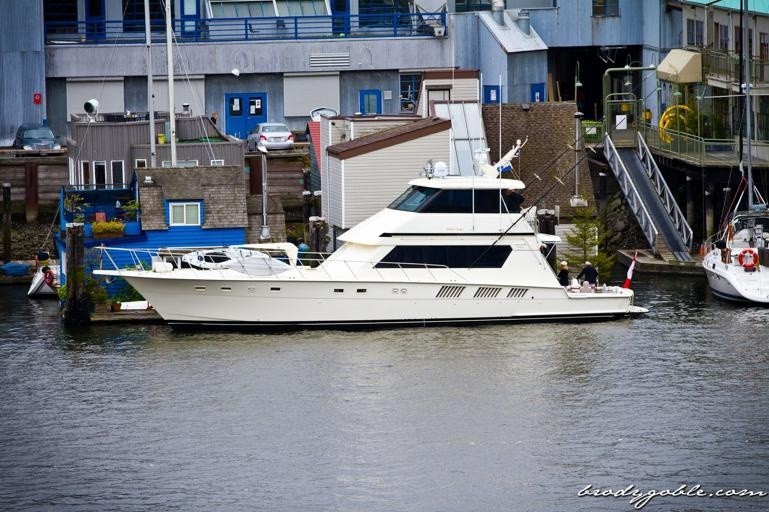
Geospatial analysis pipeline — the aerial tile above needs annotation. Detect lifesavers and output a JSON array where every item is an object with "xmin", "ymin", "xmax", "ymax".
[
  {"xmin": 44, "ymin": 270, "xmax": 54, "ymax": 286},
  {"xmin": 738, "ymin": 248, "xmax": 759, "ymax": 270}
]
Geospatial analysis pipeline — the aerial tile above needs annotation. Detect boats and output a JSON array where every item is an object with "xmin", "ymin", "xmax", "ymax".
[
  {"xmin": 93, "ymin": 15, "xmax": 650, "ymax": 332},
  {"xmin": 701, "ymin": 215, "xmax": 769, "ymax": 305}
]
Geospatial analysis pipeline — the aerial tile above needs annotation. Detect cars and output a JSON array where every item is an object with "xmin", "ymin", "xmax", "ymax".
[
  {"xmin": 247, "ymin": 122, "xmax": 295, "ymax": 151},
  {"xmin": 13, "ymin": 123, "xmax": 62, "ymax": 150}
]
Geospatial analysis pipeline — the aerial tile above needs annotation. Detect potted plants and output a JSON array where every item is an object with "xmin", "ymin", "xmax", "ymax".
[{"xmin": 92, "ymin": 222, "xmax": 125, "ymax": 239}]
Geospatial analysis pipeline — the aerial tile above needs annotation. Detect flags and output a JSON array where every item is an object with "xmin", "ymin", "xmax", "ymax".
[{"xmin": 623, "ymin": 254, "xmax": 636, "ymax": 288}]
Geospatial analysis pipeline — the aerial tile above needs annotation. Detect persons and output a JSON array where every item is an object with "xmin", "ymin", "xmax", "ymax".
[
  {"xmin": 575, "ymin": 260, "xmax": 597, "ymax": 284},
  {"xmin": 556, "ymin": 260, "xmax": 570, "ymax": 287}
]
